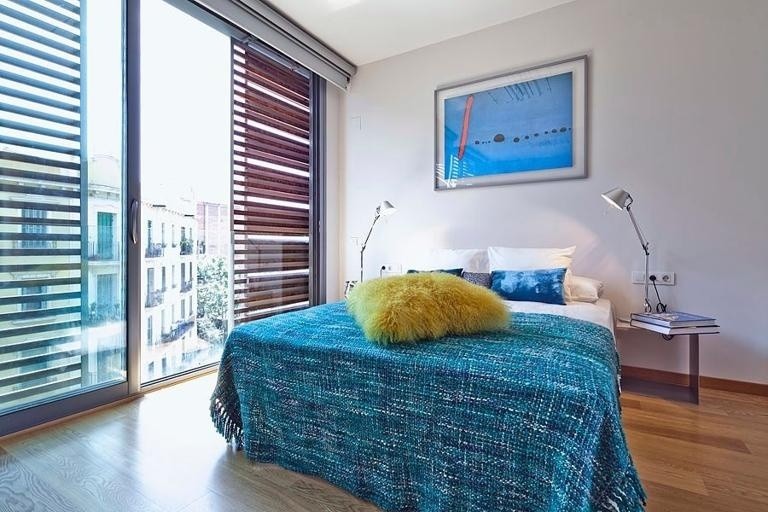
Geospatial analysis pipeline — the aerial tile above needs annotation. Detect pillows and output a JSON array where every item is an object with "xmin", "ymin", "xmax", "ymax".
[
  {"xmin": 490, "ymin": 266, "xmax": 567, "ymax": 305},
  {"xmin": 569, "ymin": 275, "xmax": 604, "ymax": 303},
  {"xmin": 407, "ymin": 268, "xmax": 461, "ymax": 276},
  {"xmin": 463, "ymin": 271, "xmax": 491, "ymax": 288},
  {"xmin": 484, "ymin": 246, "xmax": 575, "ymax": 305},
  {"xmin": 426, "ymin": 248, "xmax": 487, "ymax": 272},
  {"xmin": 346, "ymin": 269, "xmax": 514, "ymax": 347}
]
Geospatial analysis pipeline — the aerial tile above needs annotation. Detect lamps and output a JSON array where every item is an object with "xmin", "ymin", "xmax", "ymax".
[
  {"xmin": 600, "ymin": 187, "xmax": 653, "ymax": 323},
  {"xmin": 359, "ymin": 199, "xmax": 396, "ymax": 281}
]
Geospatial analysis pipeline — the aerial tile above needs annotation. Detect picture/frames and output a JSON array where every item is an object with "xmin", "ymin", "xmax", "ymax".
[{"xmin": 432, "ymin": 54, "xmax": 590, "ymax": 191}]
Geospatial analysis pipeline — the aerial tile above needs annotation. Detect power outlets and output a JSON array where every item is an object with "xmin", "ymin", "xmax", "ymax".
[{"xmin": 633, "ymin": 270, "xmax": 675, "ymax": 286}]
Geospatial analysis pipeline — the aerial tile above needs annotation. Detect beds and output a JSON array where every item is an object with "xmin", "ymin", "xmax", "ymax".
[{"xmin": 209, "ymin": 295, "xmax": 646, "ymax": 512}]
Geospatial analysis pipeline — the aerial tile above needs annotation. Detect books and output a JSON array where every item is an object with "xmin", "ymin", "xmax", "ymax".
[
  {"xmin": 629, "ymin": 319, "xmax": 720, "ymax": 337},
  {"xmin": 630, "ymin": 311, "xmax": 716, "ymax": 328}
]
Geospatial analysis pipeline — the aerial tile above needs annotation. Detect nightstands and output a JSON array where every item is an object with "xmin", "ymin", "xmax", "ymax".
[{"xmin": 617, "ymin": 321, "xmax": 699, "ymax": 405}]
